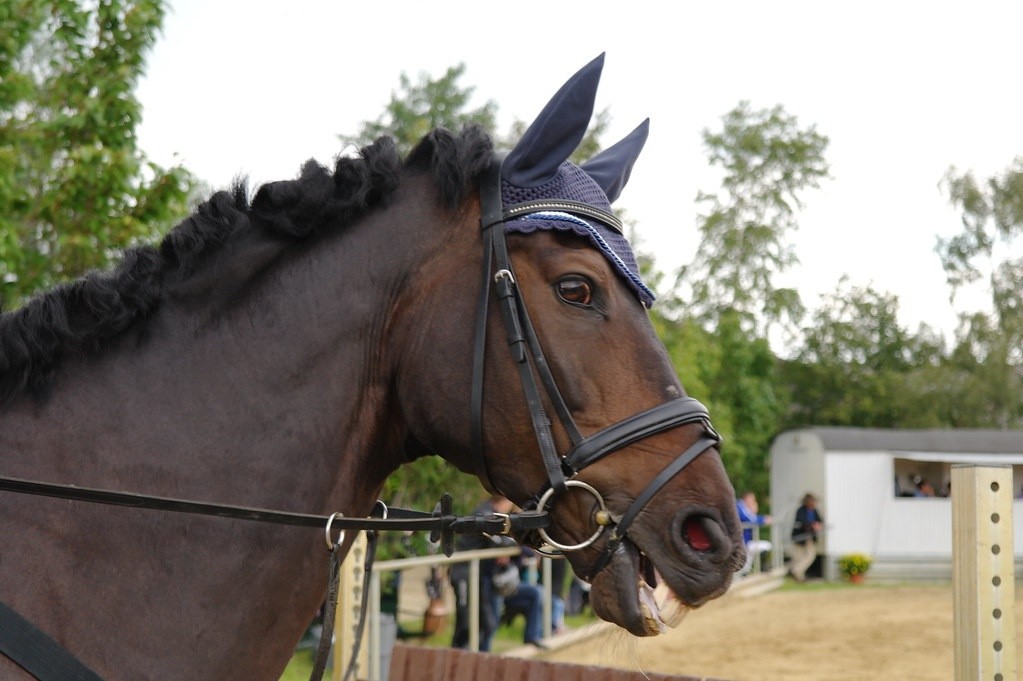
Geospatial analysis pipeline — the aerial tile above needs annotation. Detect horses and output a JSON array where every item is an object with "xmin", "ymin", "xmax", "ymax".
[{"xmin": 0, "ymin": 50, "xmax": 749, "ymax": 681}]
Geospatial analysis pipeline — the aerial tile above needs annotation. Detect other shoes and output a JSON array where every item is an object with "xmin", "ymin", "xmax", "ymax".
[{"xmin": 789, "ymin": 565, "xmax": 807, "ymax": 584}]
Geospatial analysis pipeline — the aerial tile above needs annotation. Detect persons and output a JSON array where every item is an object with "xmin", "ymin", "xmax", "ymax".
[
  {"xmin": 736, "ymin": 492, "xmax": 775, "ymax": 578},
  {"xmin": 788, "ymin": 493, "xmax": 828, "ymax": 583},
  {"xmin": 899, "ymin": 479, "xmax": 952, "ymax": 500},
  {"xmin": 454, "ymin": 487, "xmax": 590, "ymax": 651}
]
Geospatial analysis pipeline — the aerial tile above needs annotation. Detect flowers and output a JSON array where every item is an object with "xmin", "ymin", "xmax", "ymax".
[{"xmin": 839, "ymin": 551, "xmax": 871, "ymax": 574}]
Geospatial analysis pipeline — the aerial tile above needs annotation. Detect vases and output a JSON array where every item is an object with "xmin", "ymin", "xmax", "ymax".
[{"xmin": 848, "ymin": 569, "xmax": 865, "ymax": 584}]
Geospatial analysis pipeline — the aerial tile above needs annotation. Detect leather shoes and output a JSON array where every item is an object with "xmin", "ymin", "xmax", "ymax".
[{"xmin": 525, "ymin": 637, "xmax": 551, "ymax": 649}]
